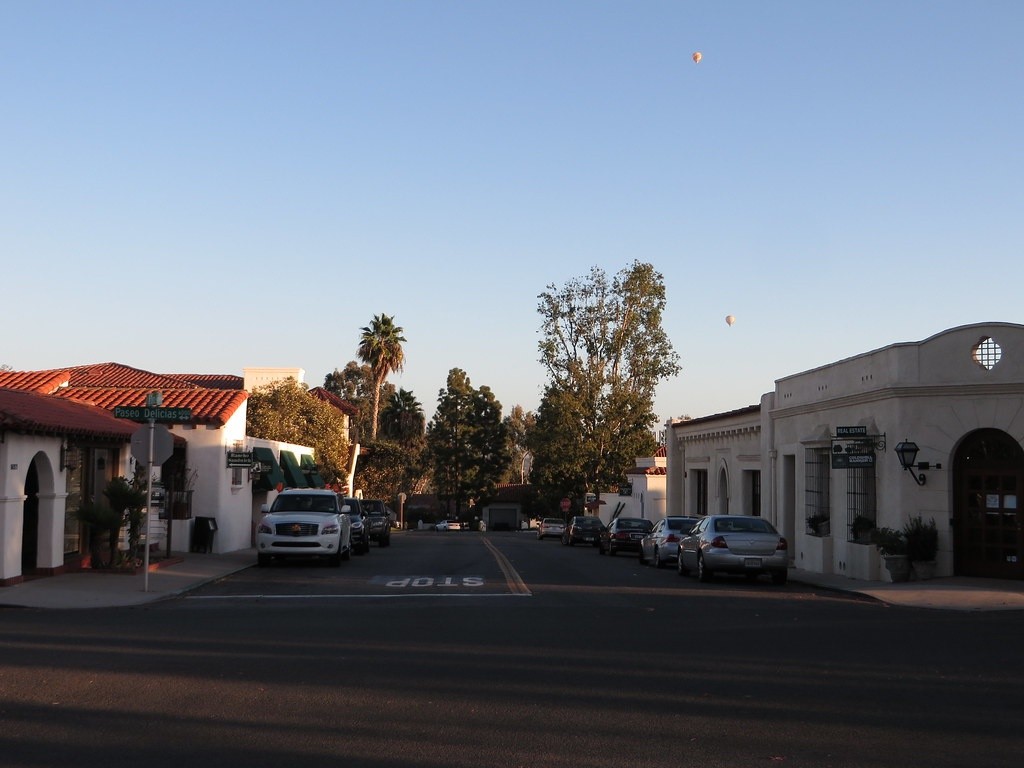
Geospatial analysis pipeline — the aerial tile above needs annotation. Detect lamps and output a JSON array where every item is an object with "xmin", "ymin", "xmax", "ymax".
[
  {"xmin": 894, "ymin": 438, "xmax": 942, "ymax": 487},
  {"xmin": 60, "ymin": 441, "xmax": 82, "ymax": 474}
]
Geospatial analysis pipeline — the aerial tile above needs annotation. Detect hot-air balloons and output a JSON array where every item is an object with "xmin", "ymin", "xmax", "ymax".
[{"xmin": 725, "ymin": 315, "xmax": 735, "ymax": 327}]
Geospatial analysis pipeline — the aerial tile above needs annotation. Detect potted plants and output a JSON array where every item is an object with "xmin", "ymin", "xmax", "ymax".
[
  {"xmin": 805, "ymin": 513, "xmax": 830, "ymax": 537},
  {"xmin": 850, "ymin": 515, "xmax": 875, "ymax": 544},
  {"xmin": 901, "ymin": 512, "xmax": 939, "ymax": 583},
  {"xmin": 870, "ymin": 527, "xmax": 912, "ymax": 584}
]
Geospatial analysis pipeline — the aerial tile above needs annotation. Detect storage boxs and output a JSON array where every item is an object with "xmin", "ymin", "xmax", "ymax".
[{"xmin": 159, "ymin": 489, "xmax": 195, "ymax": 520}]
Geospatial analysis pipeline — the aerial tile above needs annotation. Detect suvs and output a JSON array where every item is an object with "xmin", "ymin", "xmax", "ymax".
[
  {"xmin": 360, "ymin": 499, "xmax": 391, "ymax": 549},
  {"xmin": 342, "ymin": 497, "xmax": 369, "ymax": 557},
  {"xmin": 255, "ymin": 489, "xmax": 352, "ymax": 567}
]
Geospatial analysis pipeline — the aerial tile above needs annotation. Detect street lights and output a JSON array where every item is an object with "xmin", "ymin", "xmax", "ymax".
[{"xmin": 143, "ymin": 390, "xmax": 163, "ymax": 593}]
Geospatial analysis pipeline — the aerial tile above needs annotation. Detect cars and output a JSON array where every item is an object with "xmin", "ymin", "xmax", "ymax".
[
  {"xmin": 537, "ymin": 518, "xmax": 566, "ymax": 539},
  {"xmin": 392, "ymin": 520, "xmax": 401, "ymax": 528},
  {"xmin": 599, "ymin": 517, "xmax": 653, "ymax": 556},
  {"xmin": 562, "ymin": 516, "xmax": 606, "ymax": 547},
  {"xmin": 436, "ymin": 520, "xmax": 461, "ymax": 532},
  {"xmin": 638, "ymin": 515, "xmax": 703, "ymax": 569},
  {"xmin": 678, "ymin": 514, "xmax": 788, "ymax": 585}
]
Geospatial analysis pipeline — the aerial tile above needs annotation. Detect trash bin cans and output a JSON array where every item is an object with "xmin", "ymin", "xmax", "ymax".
[{"xmin": 191, "ymin": 516, "xmax": 219, "ymax": 554}]
